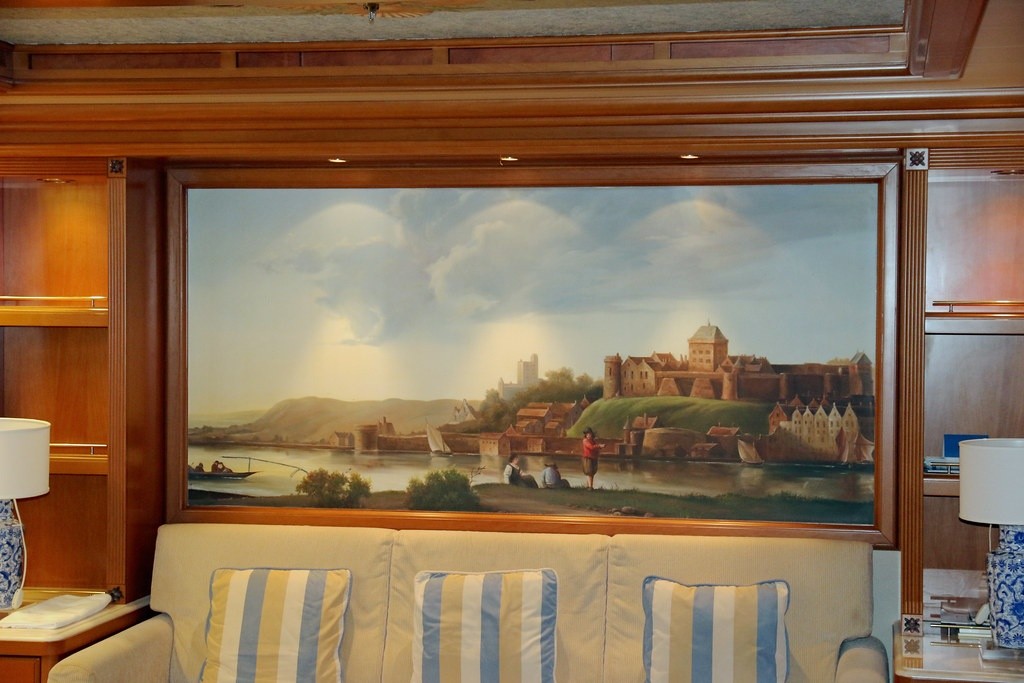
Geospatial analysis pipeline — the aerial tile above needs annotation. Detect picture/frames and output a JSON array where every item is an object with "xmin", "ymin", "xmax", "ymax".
[{"xmin": 167, "ymin": 161, "xmax": 899, "ymax": 548}]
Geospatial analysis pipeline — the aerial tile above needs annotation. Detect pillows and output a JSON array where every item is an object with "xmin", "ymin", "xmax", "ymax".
[
  {"xmin": 196, "ymin": 566, "xmax": 349, "ymax": 682},
  {"xmin": 641, "ymin": 574, "xmax": 790, "ymax": 683},
  {"xmin": 410, "ymin": 567, "xmax": 560, "ymax": 683}
]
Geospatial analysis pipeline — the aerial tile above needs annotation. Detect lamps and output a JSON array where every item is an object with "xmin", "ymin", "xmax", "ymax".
[
  {"xmin": 959, "ymin": 438, "xmax": 1023, "ymax": 647},
  {"xmin": 0, "ymin": 417, "xmax": 50, "ymax": 613}
]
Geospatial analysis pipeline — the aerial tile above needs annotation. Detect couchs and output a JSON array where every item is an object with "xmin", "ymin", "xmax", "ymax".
[{"xmin": 49, "ymin": 522, "xmax": 887, "ymax": 683}]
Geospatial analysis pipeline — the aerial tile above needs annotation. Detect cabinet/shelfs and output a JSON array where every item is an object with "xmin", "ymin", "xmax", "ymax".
[
  {"xmin": 0, "ymin": 588, "xmax": 160, "ymax": 683},
  {"xmin": 893, "ymin": 619, "xmax": 1024, "ymax": 682},
  {"xmin": 0, "ymin": 156, "xmax": 170, "ymax": 605},
  {"xmin": 897, "ymin": 149, "xmax": 1022, "ymax": 635}
]
